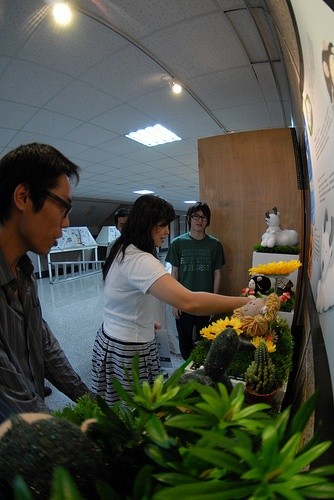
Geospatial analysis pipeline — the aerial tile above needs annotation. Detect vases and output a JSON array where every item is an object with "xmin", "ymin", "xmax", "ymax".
[{"xmin": 277, "ymin": 309, "xmax": 295, "ymax": 327}]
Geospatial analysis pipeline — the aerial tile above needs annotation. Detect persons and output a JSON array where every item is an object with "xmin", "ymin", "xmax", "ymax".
[
  {"xmin": 91, "ymin": 193, "xmax": 251, "ymax": 415},
  {"xmin": 0, "ymin": 142, "xmax": 92, "ymax": 436}
]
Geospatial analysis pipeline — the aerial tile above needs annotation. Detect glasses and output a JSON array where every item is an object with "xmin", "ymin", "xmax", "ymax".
[
  {"xmin": 45, "ymin": 190, "xmax": 72, "ymax": 217},
  {"xmin": 191, "ymin": 214, "xmax": 208, "ymax": 222}
]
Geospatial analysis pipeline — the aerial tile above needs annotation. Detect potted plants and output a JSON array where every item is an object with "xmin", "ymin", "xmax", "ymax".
[{"xmin": 246, "ymin": 340, "xmax": 280, "ymax": 406}]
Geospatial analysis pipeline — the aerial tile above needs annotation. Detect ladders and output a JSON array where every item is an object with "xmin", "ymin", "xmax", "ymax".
[{"xmin": 49, "ymin": 261, "xmax": 107, "ymax": 285}]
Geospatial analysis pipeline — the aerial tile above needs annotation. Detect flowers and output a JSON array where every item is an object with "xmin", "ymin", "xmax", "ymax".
[
  {"xmin": 200, "ymin": 318, "xmax": 278, "ymax": 354},
  {"xmin": 240, "ymin": 260, "xmax": 302, "ymax": 312}
]
